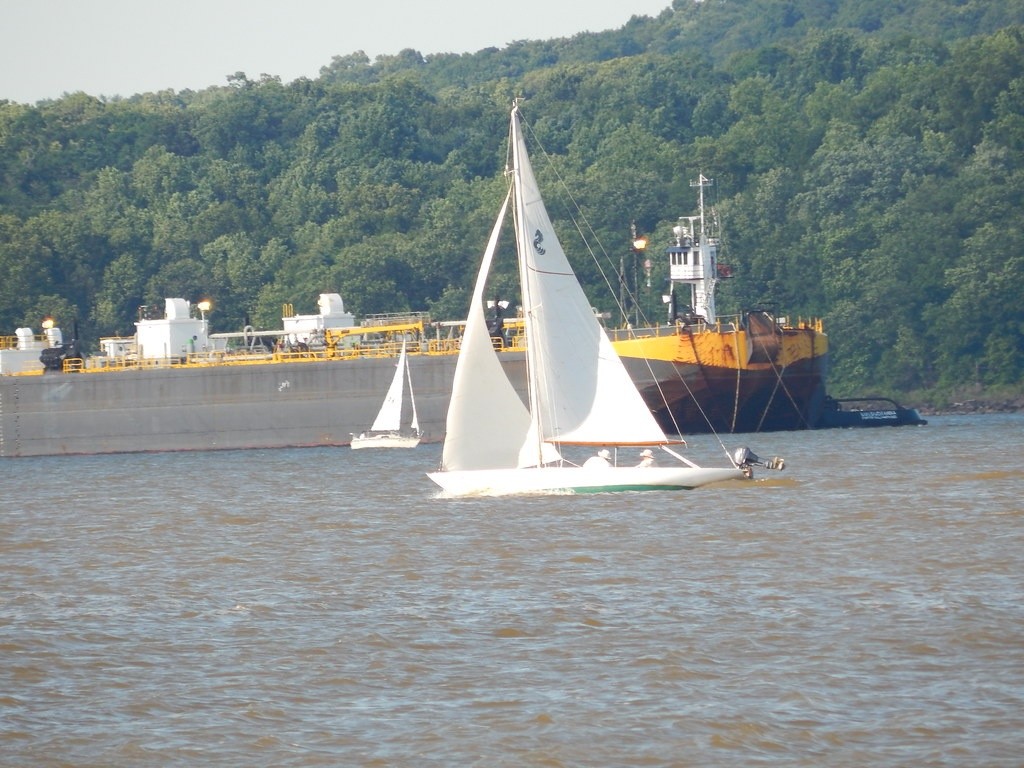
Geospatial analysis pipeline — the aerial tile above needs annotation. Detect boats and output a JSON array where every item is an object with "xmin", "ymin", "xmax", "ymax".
[{"xmin": 0, "ymin": 173, "xmax": 929, "ymax": 458}]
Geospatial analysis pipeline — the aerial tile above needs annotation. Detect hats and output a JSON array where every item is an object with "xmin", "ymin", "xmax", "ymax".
[
  {"xmin": 598, "ymin": 449, "xmax": 611, "ymax": 460},
  {"xmin": 639, "ymin": 449, "xmax": 655, "ymax": 459}
]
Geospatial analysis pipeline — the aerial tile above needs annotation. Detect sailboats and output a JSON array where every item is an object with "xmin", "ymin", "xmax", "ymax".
[
  {"xmin": 349, "ymin": 338, "xmax": 425, "ymax": 449},
  {"xmin": 425, "ymin": 98, "xmax": 786, "ymax": 497},
  {"xmin": 350, "ymin": 339, "xmax": 424, "ymax": 450}
]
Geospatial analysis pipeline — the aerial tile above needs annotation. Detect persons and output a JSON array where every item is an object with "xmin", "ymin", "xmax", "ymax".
[
  {"xmin": 635, "ymin": 449, "xmax": 656, "ymax": 468},
  {"xmin": 583, "ymin": 449, "xmax": 614, "ymax": 468}
]
